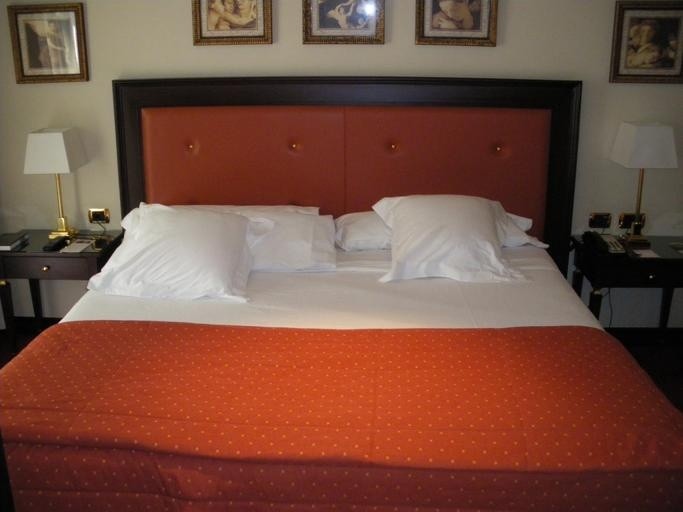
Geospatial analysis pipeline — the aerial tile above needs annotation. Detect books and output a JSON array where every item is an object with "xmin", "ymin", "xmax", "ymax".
[{"xmin": 0, "ymin": 232, "xmax": 30, "ymax": 252}]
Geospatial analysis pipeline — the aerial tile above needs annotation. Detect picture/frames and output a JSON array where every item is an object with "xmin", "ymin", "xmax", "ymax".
[
  {"xmin": 5, "ymin": 3, "xmax": 90, "ymax": 83},
  {"xmin": 608, "ymin": 0, "xmax": 683, "ymax": 84}
]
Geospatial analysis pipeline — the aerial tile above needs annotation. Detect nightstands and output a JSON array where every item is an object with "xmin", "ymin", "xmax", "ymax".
[
  {"xmin": 1, "ymin": 230, "xmax": 123, "ymax": 353},
  {"xmin": 571, "ymin": 235, "xmax": 683, "ymax": 343}
]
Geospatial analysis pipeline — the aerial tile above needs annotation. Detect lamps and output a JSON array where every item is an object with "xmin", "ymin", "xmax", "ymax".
[
  {"xmin": 607, "ymin": 121, "xmax": 678, "ymax": 249},
  {"xmin": 23, "ymin": 127, "xmax": 91, "ymax": 243}
]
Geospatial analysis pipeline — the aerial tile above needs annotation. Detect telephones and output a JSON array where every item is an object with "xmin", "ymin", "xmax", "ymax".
[{"xmin": 582, "ymin": 231, "xmax": 626, "ymax": 255}]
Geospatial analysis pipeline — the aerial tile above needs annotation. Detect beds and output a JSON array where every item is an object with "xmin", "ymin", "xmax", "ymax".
[{"xmin": 1, "ymin": 77, "xmax": 681, "ymax": 510}]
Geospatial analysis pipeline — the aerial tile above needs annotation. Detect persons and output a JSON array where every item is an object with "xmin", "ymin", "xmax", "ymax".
[
  {"xmin": 204, "ymin": 0, "xmax": 483, "ymax": 32},
  {"xmin": 626, "ymin": 21, "xmax": 670, "ymax": 68},
  {"xmin": 43, "ymin": 23, "xmax": 65, "ymax": 72}
]
